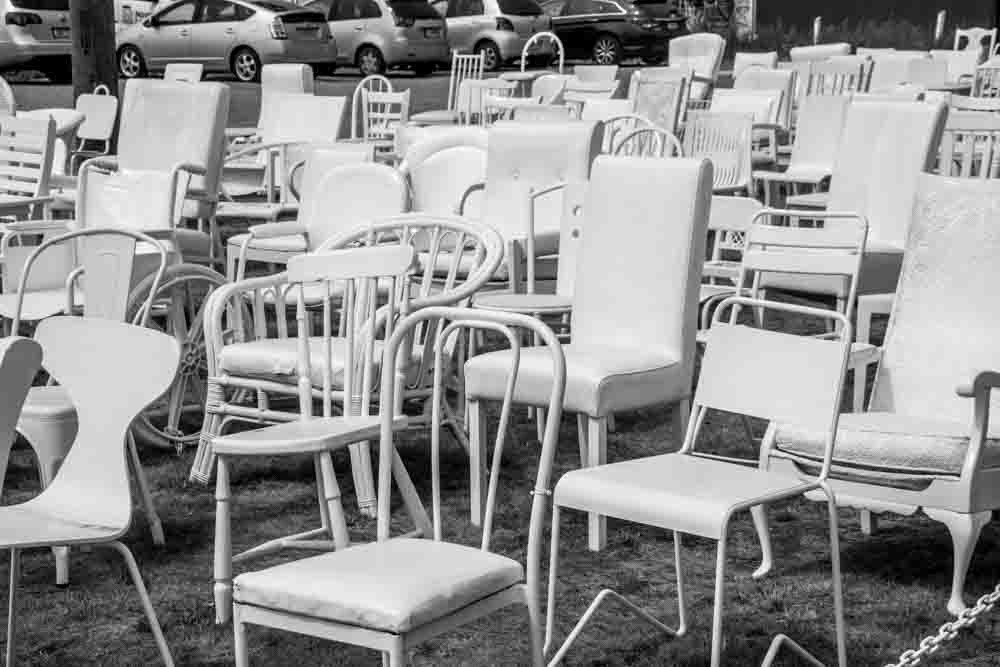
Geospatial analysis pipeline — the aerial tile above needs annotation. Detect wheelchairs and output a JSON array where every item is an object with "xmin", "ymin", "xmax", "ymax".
[{"xmin": 1, "ymin": 157, "xmax": 255, "ymax": 456}]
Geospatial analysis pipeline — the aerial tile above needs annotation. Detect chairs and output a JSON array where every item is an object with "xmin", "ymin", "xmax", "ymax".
[
  {"xmin": 204, "ymin": 6, "xmax": 221, "ymax": 21},
  {"xmin": 0, "ymin": 23, "xmax": 1000, "ymax": 667}
]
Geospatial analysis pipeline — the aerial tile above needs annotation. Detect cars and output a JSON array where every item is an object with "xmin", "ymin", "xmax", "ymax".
[
  {"xmin": 116, "ymin": 0, "xmax": 337, "ymax": 82},
  {"xmin": 428, "ymin": 0, "xmax": 556, "ymax": 70},
  {"xmin": 538, "ymin": 0, "xmax": 688, "ymax": 66},
  {"xmin": 302, "ymin": 0, "xmax": 448, "ymax": 76}
]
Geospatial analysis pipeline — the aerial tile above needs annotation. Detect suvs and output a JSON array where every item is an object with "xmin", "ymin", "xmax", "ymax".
[{"xmin": 0, "ymin": 0, "xmax": 73, "ymax": 83}]
